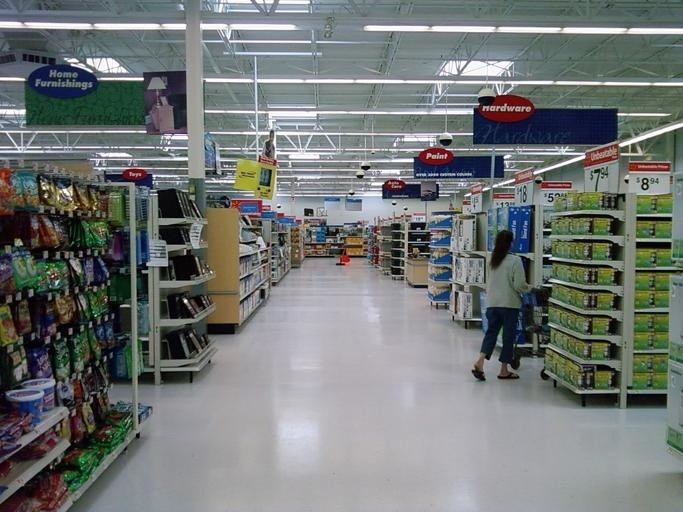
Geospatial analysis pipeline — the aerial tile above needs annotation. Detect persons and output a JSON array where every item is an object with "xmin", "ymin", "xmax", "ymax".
[
  {"xmin": 471, "ymin": 230, "xmax": 534, "ymax": 380},
  {"xmin": 334, "ymin": 228, "xmax": 339, "ymax": 236},
  {"xmin": 326, "ymin": 228, "xmax": 330, "ymax": 236},
  {"xmin": 262, "ymin": 130, "xmax": 275, "ymax": 159}
]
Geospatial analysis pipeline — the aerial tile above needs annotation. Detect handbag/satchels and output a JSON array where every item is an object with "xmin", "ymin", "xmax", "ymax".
[{"xmin": 509, "ymin": 350, "xmax": 522, "ymax": 370}]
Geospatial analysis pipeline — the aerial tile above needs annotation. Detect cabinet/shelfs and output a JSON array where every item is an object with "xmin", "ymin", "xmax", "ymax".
[
  {"xmin": 303, "ymin": 219, "xmax": 363, "ymax": 257},
  {"xmin": 428, "ymin": 212, "xmax": 452, "ymax": 310},
  {"xmin": 541, "ymin": 191, "xmax": 669, "ymax": 408},
  {"xmin": 447, "ymin": 206, "xmax": 491, "ymax": 330},
  {"xmin": 389, "ymin": 219, "xmax": 427, "ymax": 287},
  {"xmin": 479, "ymin": 205, "xmax": 549, "ymax": 360},
  {"xmin": 133, "ymin": 183, "xmax": 305, "ymax": 387},
  {"xmin": 366, "ymin": 224, "xmax": 389, "ymax": 276}
]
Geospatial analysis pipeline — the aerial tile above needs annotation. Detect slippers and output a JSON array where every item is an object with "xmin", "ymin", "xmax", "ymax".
[
  {"xmin": 497, "ymin": 372, "xmax": 520, "ymax": 379},
  {"xmin": 472, "ymin": 368, "xmax": 486, "ymax": 381}
]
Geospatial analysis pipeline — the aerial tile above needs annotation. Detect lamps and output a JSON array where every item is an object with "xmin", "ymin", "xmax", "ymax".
[
  {"xmin": 356, "ymin": 122, "xmax": 371, "ymax": 179},
  {"xmin": 438, "ymin": 81, "xmax": 454, "ymax": 146},
  {"xmin": 477, "ymin": 33, "xmax": 497, "ymax": 107}
]
